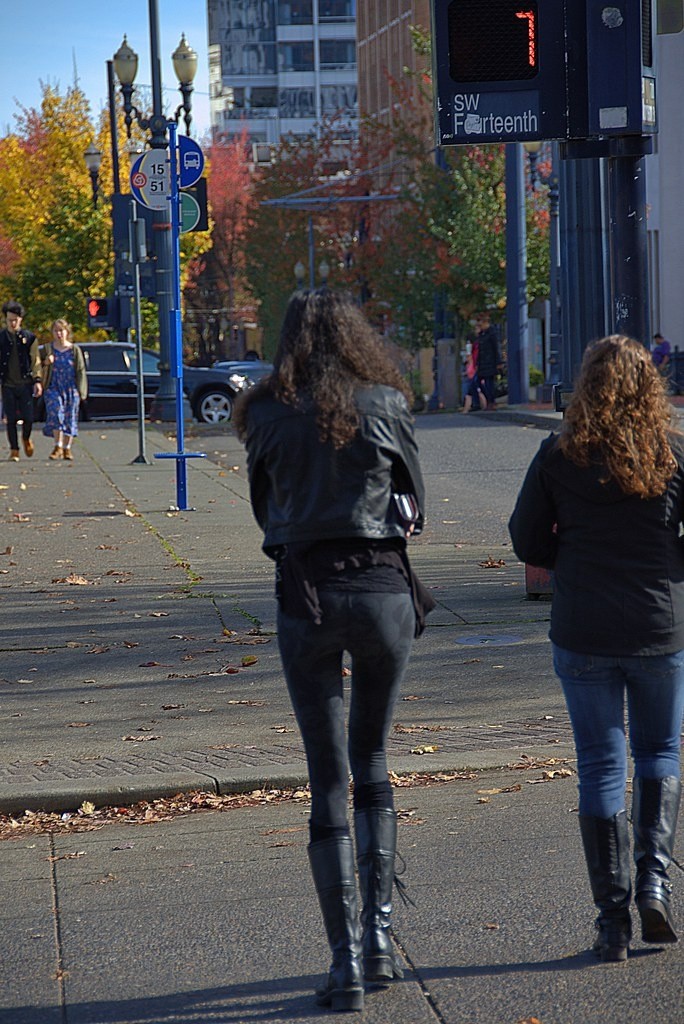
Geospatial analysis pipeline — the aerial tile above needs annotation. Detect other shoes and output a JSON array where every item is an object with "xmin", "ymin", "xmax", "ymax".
[
  {"xmin": 63, "ymin": 448, "xmax": 74, "ymax": 460},
  {"xmin": 8, "ymin": 450, "xmax": 20, "ymax": 462},
  {"xmin": 21, "ymin": 432, "xmax": 34, "ymax": 457},
  {"xmin": 48, "ymin": 445, "xmax": 64, "ymax": 459}
]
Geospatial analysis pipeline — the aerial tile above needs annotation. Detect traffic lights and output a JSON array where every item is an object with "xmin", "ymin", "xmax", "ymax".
[
  {"xmin": 85, "ymin": 296, "xmax": 109, "ymax": 330},
  {"xmin": 430, "ymin": 1, "xmax": 572, "ymax": 145}
]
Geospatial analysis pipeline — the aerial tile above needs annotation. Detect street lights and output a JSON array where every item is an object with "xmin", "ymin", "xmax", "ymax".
[
  {"xmin": 78, "ymin": 137, "xmax": 154, "ymax": 467},
  {"xmin": 110, "ymin": 31, "xmax": 212, "ymax": 423}
]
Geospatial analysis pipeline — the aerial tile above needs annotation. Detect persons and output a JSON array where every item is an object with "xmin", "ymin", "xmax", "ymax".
[
  {"xmin": 460, "ymin": 311, "xmax": 503, "ymax": 414},
  {"xmin": 182, "ymin": 345, "xmax": 201, "ymax": 368},
  {"xmin": 231, "ymin": 286, "xmax": 437, "ymax": 1011},
  {"xmin": 42, "ymin": 319, "xmax": 87, "ymax": 461},
  {"xmin": 0, "ymin": 301, "xmax": 42, "ymax": 462},
  {"xmin": 507, "ymin": 333, "xmax": 684, "ymax": 961}
]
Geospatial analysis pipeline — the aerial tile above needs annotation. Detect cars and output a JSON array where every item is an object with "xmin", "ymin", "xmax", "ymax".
[
  {"xmin": 28, "ymin": 341, "xmax": 257, "ymax": 424},
  {"xmin": 216, "ymin": 363, "xmax": 276, "ymax": 386}
]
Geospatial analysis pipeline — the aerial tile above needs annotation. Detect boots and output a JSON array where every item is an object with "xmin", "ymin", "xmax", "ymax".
[
  {"xmin": 352, "ymin": 806, "xmax": 419, "ymax": 989},
  {"xmin": 632, "ymin": 776, "xmax": 682, "ymax": 944},
  {"xmin": 306, "ymin": 836, "xmax": 365, "ymax": 1011},
  {"xmin": 578, "ymin": 809, "xmax": 631, "ymax": 961}
]
